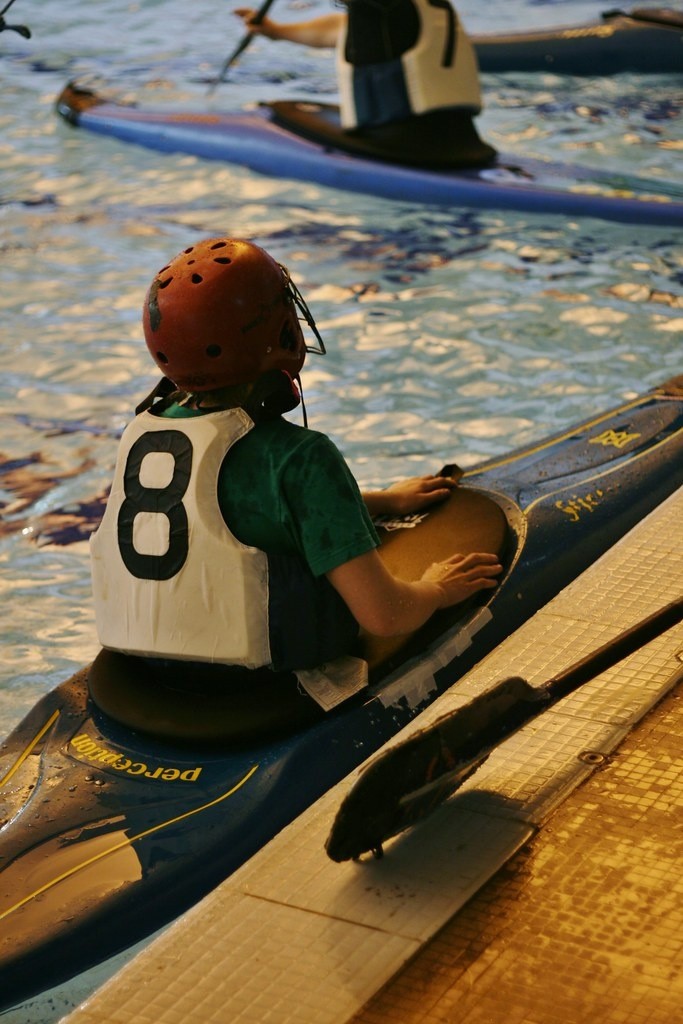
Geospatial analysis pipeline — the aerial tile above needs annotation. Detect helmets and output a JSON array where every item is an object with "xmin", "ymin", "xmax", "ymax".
[{"xmin": 141, "ymin": 237, "xmax": 307, "ymax": 392}]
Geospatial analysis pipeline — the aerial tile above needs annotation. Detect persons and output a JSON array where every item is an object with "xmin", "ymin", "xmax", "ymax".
[
  {"xmin": 91, "ymin": 239, "xmax": 503, "ymax": 698},
  {"xmin": 232, "ymin": 0, "xmax": 497, "ymax": 170}
]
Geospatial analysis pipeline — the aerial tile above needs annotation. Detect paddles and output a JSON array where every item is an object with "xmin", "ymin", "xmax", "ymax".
[
  {"xmin": 324, "ymin": 596, "xmax": 683, "ymax": 864},
  {"xmin": 205, "ymin": 0, "xmax": 275, "ymax": 96},
  {"xmin": 601, "ymin": 8, "xmax": 683, "ymax": 28}
]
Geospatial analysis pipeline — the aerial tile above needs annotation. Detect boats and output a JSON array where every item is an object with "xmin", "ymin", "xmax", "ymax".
[
  {"xmin": 467, "ymin": 10, "xmax": 683, "ymax": 89},
  {"xmin": 0, "ymin": 379, "xmax": 683, "ymax": 1024},
  {"xmin": 44, "ymin": 67, "xmax": 683, "ymax": 250}
]
